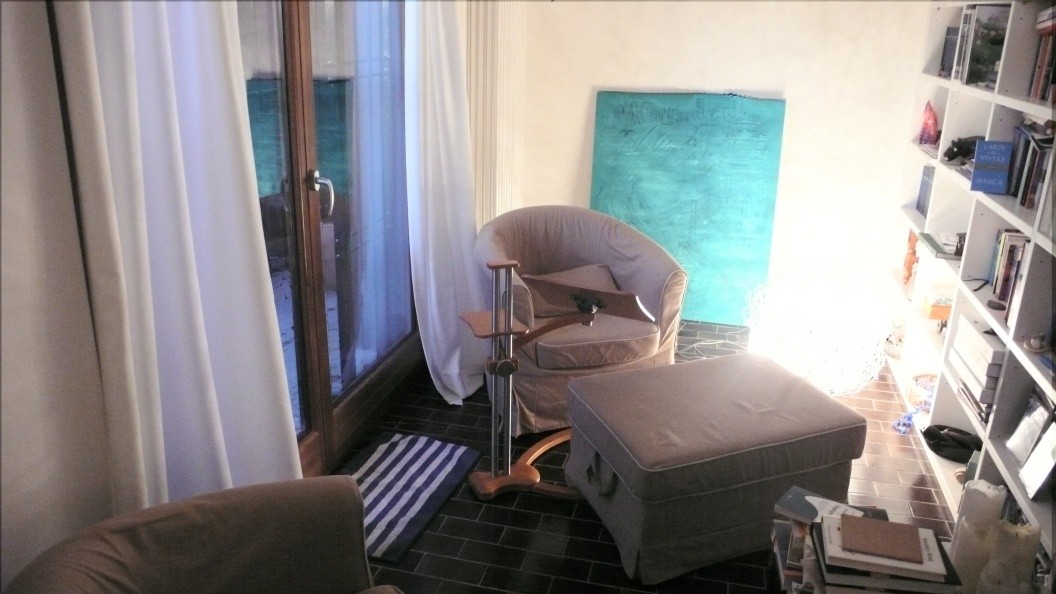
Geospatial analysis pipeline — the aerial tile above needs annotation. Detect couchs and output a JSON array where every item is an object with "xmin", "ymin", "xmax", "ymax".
[
  {"xmin": 0, "ymin": 475, "xmax": 405, "ymax": 594},
  {"xmin": 470, "ymin": 205, "xmax": 687, "ymax": 437}
]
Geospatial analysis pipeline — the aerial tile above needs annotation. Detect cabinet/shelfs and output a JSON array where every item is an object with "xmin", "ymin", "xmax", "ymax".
[{"xmin": 887, "ymin": 0, "xmax": 1056, "ymax": 594}]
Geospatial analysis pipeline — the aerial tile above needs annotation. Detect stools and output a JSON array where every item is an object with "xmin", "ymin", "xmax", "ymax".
[{"xmin": 563, "ymin": 351, "xmax": 872, "ymax": 587}]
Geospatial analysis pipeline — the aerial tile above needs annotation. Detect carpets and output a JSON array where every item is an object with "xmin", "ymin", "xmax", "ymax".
[{"xmin": 348, "ymin": 430, "xmax": 482, "ymax": 564}]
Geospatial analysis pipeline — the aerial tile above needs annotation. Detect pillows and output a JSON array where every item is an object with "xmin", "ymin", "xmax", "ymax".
[{"xmin": 526, "ymin": 265, "xmax": 619, "ymax": 319}]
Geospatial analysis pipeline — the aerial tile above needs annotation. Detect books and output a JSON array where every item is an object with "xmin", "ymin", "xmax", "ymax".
[
  {"xmin": 970, "ymin": 126, "xmax": 1053, "ymax": 213},
  {"xmin": 938, "ymin": 4, "xmax": 1056, "ymax": 110},
  {"xmin": 987, "ymin": 228, "xmax": 1031, "ymax": 329},
  {"xmin": 772, "ymin": 484, "xmax": 963, "ymax": 594}
]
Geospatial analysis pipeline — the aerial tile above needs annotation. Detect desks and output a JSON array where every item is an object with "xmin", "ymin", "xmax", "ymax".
[{"xmin": 461, "ymin": 261, "xmax": 655, "ymax": 506}]
